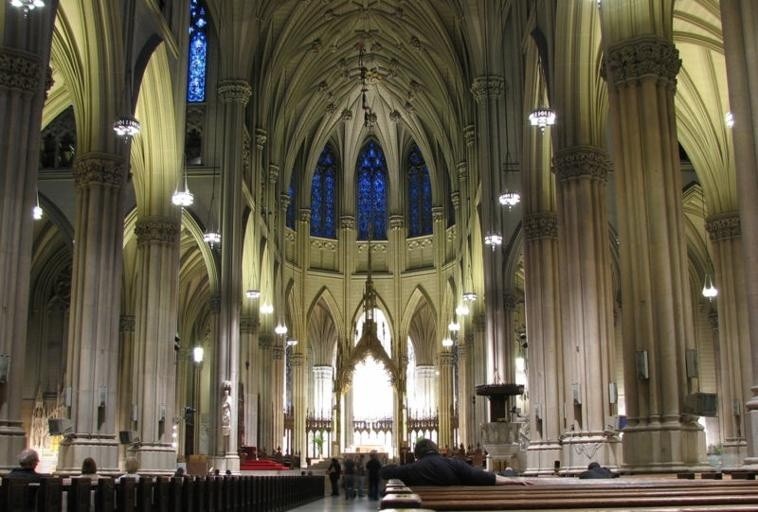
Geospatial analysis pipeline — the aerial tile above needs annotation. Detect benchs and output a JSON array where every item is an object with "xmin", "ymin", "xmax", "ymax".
[
  {"xmin": 381, "ymin": 478, "xmax": 752, "ymax": 511},
  {"xmin": 1, "ymin": 475, "xmax": 327, "ymax": 512}
]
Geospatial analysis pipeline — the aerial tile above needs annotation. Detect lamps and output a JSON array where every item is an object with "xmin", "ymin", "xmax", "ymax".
[
  {"xmin": 448, "ymin": 309, "xmax": 461, "ymax": 332},
  {"xmin": 241, "ymin": 261, "xmax": 263, "ymax": 300},
  {"xmin": 456, "ymin": 288, "xmax": 470, "ymax": 317},
  {"xmin": 461, "ymin": 262, "xmax": 477, "ymax": 303},
  {"xmin": 9, "ymin": 0, "xmax": 46, "ymax": 18},
  {"xmin": 499, "ymin": 0, "xmax": 522, "ymax": 210},
  {"xmin": 529, "ymin": 1, "xmax": 556, "ymax": 134},
  {"xmin": 171, "ymin": 0, "xmax": 195, "ymax": 205},
  {"xmin": 259, "ymin": 280, "xmax": 274, "ymax": 314},
  {"xmin": 112, "ymin": 0, "xmax": 140, "ymax": 138},
  {"xmin": 32, "ymin": 184, "xmax": 42, "ymax": 220},
  {"xmin": 202, "ymin": 0, "xmax": 224, "ymax": 246},
  {"xmin": 441, "ymin": 324, "xmax": 455, "ymax": 348},
  {"xmin": 286, "ymin": 337, "xmax": 299, "ymax": 349},
  {"xmin": 192, "ymin": 250, "xmax": 205, "ymax": 362},
  {"xmin": 701, "ymin": 189, "xmax": 718, "ymax": 302},
  {"xmin": 272, "ymin": 302, "xmax": 288, "ymax": 336},
  {"xmin": 485, "ymin": 0, "xmax": 504, "ymax": 251}
]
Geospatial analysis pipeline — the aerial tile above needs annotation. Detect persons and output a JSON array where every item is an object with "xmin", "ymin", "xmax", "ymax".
[
  {"xmin": 174, "ymin": 467, "xmax": 189, "ymax": 479},
  {"xmin": 356, "ymin": 454, "xmax": 367, "ymax": 497},
  {"xmin": 382, "ymin": 439, "xmax": 533, "ymax": 486},
  {"xmin": 77, "ymin": 456, "xmax": 102, "ymax": 480},
  {"xmin": 219, "ymin": 387, "xmax": 233, "ymax": 456},
  {"xmin": 328, "ymin": 458, "xmax": 341, "ymax": 496},
  {"xmin": 224, "ymin": 470, "xmax": 234, "ymax": 480},
  {"xmin": 579, "ymin": 462, "xmax": 619, "ymax": 478},
  {"xmin": 498, "ymin": 466, "xmax": 518, "ymax": 476},
  {"xmin": 366, "ymin": 453, "xmax": 381, "ymax": 500},
  {"xmin": 213, "ymin": 469, "xmax": 224, "ymax": 480},
  {"xmin": 9, "ymin": 448, "xmax": 45, "ymax": 482},
  {"xmin": 342, "ymin": 456, "xmax": 356, "ymax": 500},
  {"xmin": 118, "ymin": 457, "xmax": 143, "ymax": 483}
]
